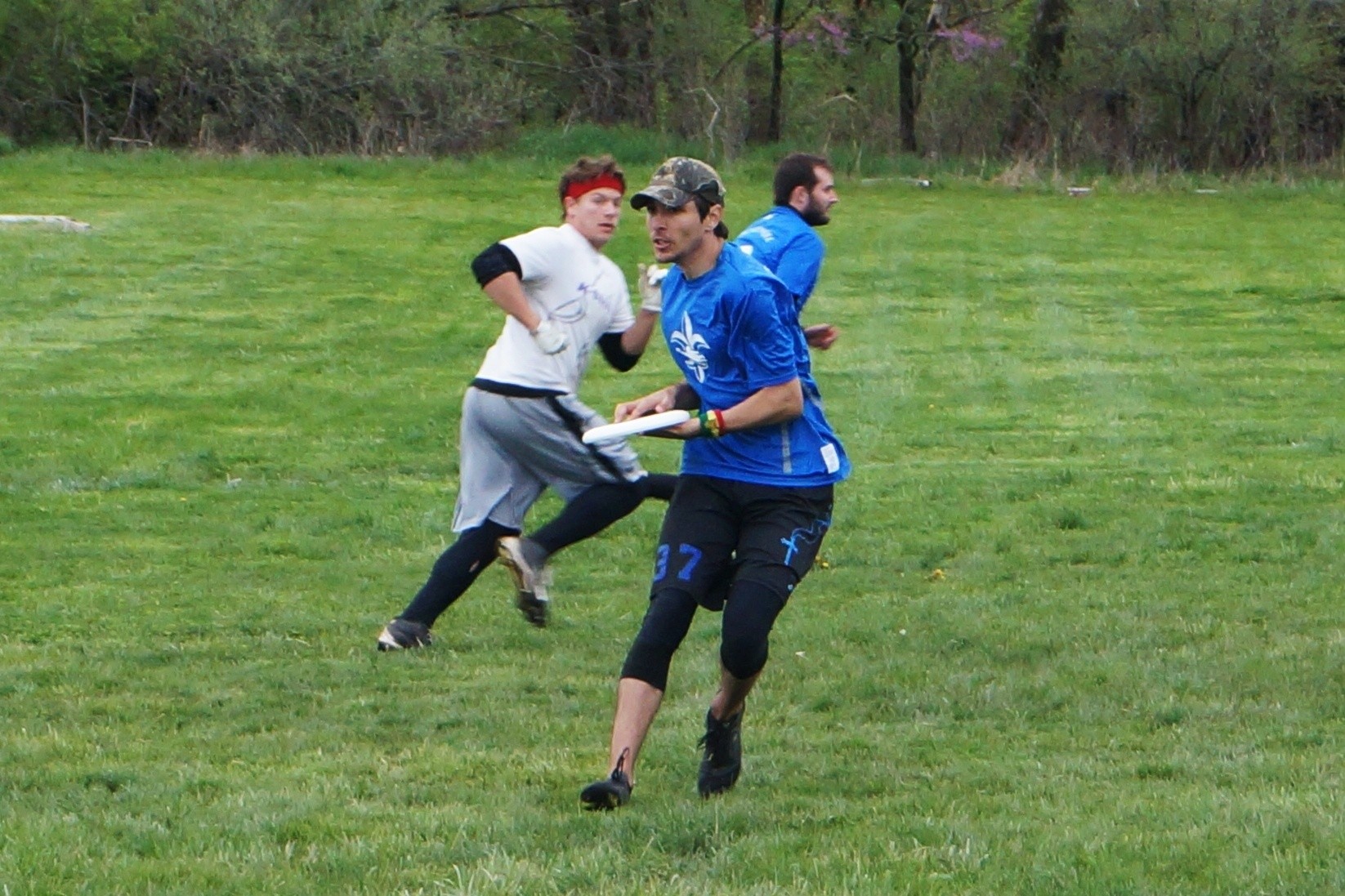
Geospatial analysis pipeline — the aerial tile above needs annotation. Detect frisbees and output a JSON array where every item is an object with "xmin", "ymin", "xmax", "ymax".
[{"xmin": 582, "ymin": 408, "xmax": 690, "ymax": 444}]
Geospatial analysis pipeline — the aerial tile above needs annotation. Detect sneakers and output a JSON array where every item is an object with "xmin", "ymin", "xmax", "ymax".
[
  {"xmin": 376, "ymin": 615, "xmax": 433, "ymax": 654},
  {"xmin": 494, "ymin": 535, "xmax": 550, "ymax": 629},
  {"xmin": 578, "ymin": 747, "xmax": 633, "ymax": 810},
  {"xmin": 695, "ymin": 688, "xmax": 748, "ymax": 803}
]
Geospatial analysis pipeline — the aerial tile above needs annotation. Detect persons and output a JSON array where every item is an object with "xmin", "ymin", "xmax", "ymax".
[
  {"xmin": 378, "ymin": 155, "xmax": 668, "ymax": 653},
  {"xmin": 647, "ymin": 153, "xmax": 839, "ymax": 497},
  {"xmin": 581, "ymin": 156, "xmax": 850, "ymax": 811}
]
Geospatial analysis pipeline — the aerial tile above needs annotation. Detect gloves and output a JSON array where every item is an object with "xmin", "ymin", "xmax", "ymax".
[
  {"xmin": 635, "ymin": 262, "xmax": 669, "ymax": 313},
  {"xmin": 529, "ymin": 316, "xmax": 570, "ymax": 357}
]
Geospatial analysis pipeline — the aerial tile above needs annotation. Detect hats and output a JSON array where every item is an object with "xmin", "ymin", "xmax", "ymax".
[{"xmin": 631, "ymin": 156, "xmax": 727, "ymax": 211}]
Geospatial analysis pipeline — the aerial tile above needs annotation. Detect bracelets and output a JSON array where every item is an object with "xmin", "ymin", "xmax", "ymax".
[{"xmin": 699, "ymin": 409, "xmax": 726, "ymax": 439}]
